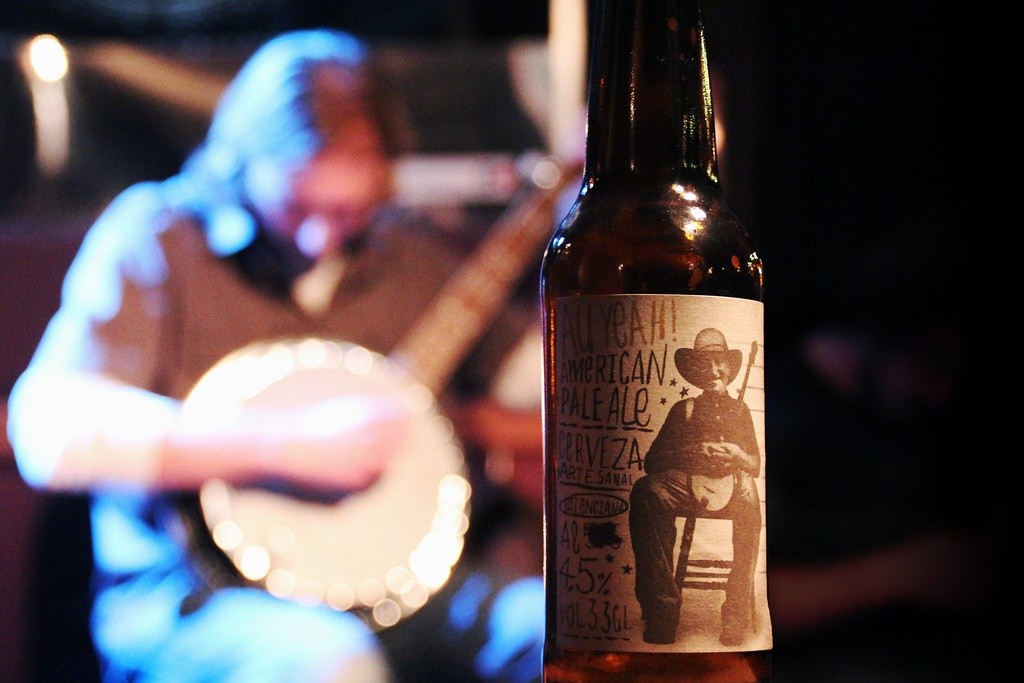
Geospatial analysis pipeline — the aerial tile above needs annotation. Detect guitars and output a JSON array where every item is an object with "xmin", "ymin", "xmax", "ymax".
[{"xmin": 178, "ymin": 153, "xmax": 580, "ymax": 633}]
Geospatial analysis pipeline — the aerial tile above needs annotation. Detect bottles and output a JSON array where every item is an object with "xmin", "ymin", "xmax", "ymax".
[{"xmin": 541, "ymin": 0, "xmax": 778, "ymax": 683}]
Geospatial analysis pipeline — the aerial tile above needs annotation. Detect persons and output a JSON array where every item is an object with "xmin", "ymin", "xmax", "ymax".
[
  {"xmin": 7, "ymin": 32, "xmax": 543, "ymax": 683},
  {"xmin": 625, "ymin": 329, "xmax": 761, "ymax": 647}
]
